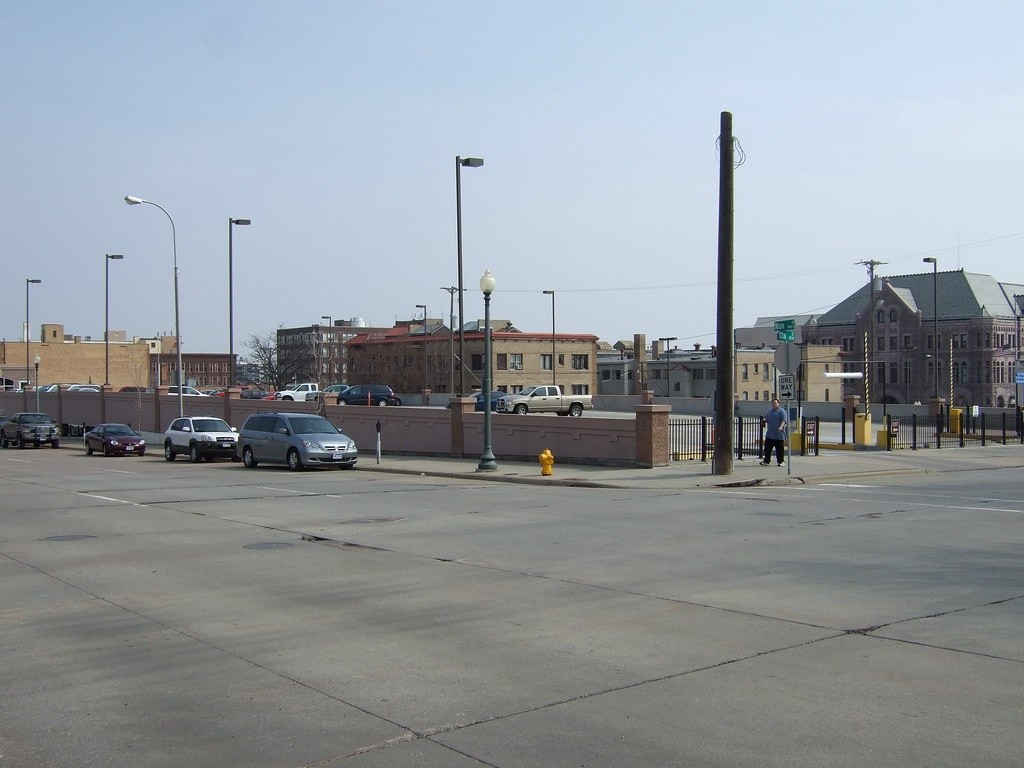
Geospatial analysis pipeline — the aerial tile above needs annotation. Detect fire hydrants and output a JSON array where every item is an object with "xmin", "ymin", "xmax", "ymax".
[{"xmin": 539, "ymin": 448, "xmax": 555, "ymax": 475}]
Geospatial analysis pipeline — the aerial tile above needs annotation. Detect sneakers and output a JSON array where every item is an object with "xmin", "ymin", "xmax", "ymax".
[
  {"xmin": 777, "ymin": 461, "xmax": 786, "ymax": 467},
  {"xmin": 759, "ymin": 461, "xmax": 770, "ymax": 466}
]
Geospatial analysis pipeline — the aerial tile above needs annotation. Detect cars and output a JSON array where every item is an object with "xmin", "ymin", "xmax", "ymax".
[
  {"xmin": 12, "ymin": 380, "xmax": 399, "ymax": 407},
  {"xmin": 447, "ymin": 390, "xmax": 509, "ymax": 412},
  {"xmin": 84, "ymin": 423, "xmax": 146, "ymax": 457}
]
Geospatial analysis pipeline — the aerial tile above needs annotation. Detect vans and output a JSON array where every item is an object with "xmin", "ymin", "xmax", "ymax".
[{"xmin": 237, "ymin": 410, "xmax": 359, "ymax": 470}]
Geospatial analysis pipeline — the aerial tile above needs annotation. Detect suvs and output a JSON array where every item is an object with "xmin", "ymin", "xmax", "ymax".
[{"xmin": 163, "ymin": 415, "xmax": 241, "ymax": 463}]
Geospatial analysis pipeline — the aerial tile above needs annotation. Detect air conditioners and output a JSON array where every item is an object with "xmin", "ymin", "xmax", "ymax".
[{"xmin": 515, "ymin": 364, "xmax": 523, "ymax": 370}]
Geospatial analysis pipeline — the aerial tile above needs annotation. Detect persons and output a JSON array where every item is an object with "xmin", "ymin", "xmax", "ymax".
[{"xmin": 760, "ymin": 398, "xmax": 788, "ymax": 467}]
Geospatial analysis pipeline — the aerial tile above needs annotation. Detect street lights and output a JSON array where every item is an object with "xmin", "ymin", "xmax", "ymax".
[
  {"xmin": 33, "ymin": 352, "xmax": 41, "ymax": 413},
  {"xmin": 923, "ymin": 256, "xmax": 939, "ymax": 399},
  {"xmin": 543, "ymin": 290, "xmax": 557, "ymax": 386},
  {"xmin": 454, "ymin": 154, "xmax": 485, "ymax": 397},
  {"xmin": 479, "ymin": 269, "xmax": 496, "ymax": 470},
  {"xmin": 105, "ymin": 253, "xmax": 124, "ymax": 385},
  {"xmin": 26, "ymin": 279, "xmax": 42, "ymax": 386},
  {"xmin": 415, "ymin": 304, "xmax": 428, "ymax": 388},
  {"xmin": 123, "ymin": 194, "xmax": 185, "ymax": 417},
  {"xmin": 228, "ymin": 216, "xmax": 252, "ymax": 388},
  {"xmin": 321, "ymin": 316, "xmax": 333, "ymax": 382}
]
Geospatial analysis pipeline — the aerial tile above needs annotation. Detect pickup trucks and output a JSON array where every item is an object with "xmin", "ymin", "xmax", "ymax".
[
  {"xmin": 0, "ymin": 412, "xmax": 61, "ymax": 450},
  {"xmin": 494, "ymin": 384, "xmax": 595, "ymax": 417}
]
